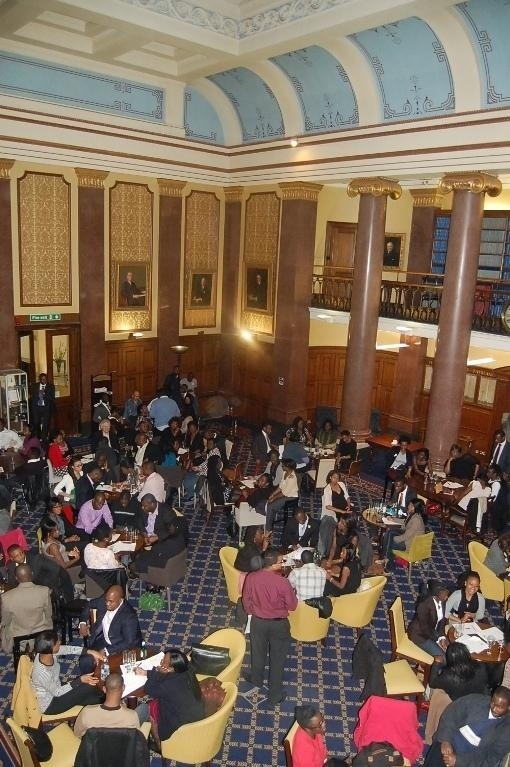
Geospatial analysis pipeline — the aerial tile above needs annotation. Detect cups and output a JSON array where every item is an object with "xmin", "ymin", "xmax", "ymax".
[
  {"xmin": 454, "ymin": 623, "xmax": 462, "ymax": 638},
  {"xmin": 365, "ymin": 506, "xmax": 371, "ymax": 519},
  {"xmin": 122, "ymin": 651, "xmax": 136, "ymax": 668},
  {"xmin": 128, "ymin": 527, "xmax": 139, "ymax": 540},
  {"xmin": 487, "ymin": 637, "xmax": 496, "ymax": 656},
  {"xmin": 124, "ymin": 695, "xmax": 137, "ymax": 709}
]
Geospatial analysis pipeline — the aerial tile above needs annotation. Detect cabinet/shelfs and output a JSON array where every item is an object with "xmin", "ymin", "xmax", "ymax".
[{"xmin": 0, "ymin": 369, "xmax": 29, "ymax": 429}]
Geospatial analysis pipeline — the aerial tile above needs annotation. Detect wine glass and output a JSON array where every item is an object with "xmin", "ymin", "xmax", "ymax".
[{"xmin": 1, "ymin": 445, "xmax": 5, "ymax": 456}]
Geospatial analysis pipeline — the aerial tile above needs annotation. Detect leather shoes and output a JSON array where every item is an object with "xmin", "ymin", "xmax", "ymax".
[
  {"xmin": 270, "ymin": 692, "xmax": 287, "ymax": 705},
  {"xmin": 243, "ymin": 672, "xmax": 264, "ymax": 688}
]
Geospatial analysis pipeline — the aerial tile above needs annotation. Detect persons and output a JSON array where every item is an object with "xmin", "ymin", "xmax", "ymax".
[
  {"xmin": 134, "ymin": 648, "xmax": 207, "ymax": 752},
  {"xmin": 242, "ymin": 548, "xmax": 298, "ymax": 707},
  {"xmin": 1, "ymin": 373, "xmax": 510, "ymax": 657},
  {"xmin": 407, "ymin": 582, "xmax": 452, "ymax": 705},
  {"xmin": 431, "ymin": 642, "xmax": 493, "ymax": 702},
  {"xmin": 292, "ymin": 706, "xmax": 330, "ymax": 767},
  {"xmin": 424, "ymin": 686, "xmax": 509, "ymax": 767},
  {"xmin": 192, "ymin": 277, "xmax": 210, "ymax": 305},
  {"xmin": 122, "ymin": 271, "xmax": 145, "ymax": 305},
  {"xmin": 74, "ymin": 673, "xmax": 150, "ymax": 740},
  {"xmin": 31, "ymin": 631, "xmax": 106, "ymax": 715},
  {"xmin": 247, "ymin": 273, "xmax": 267, "ymax": 310},
  {"xmin": 383, "ymin": 241, "xmax": 399, "ymax": 266}
]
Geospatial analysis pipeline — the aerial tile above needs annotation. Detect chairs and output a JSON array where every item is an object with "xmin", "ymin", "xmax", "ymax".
[
  {"xmin": 6, "ymin": 719, "xmax": 82, "ymax": 767},
  {"xmin": 284, "ymin": 720, "xmax": 299, "ymax": 767},
  {"xmin": 186, "ymin": 628, "xmax": 245, "ymax": 690},
  {"xmin": 388, "ymin": 596, "xmax": 434, "ymax": 688},
  {"xmin": 12, "ymin": 655, "xmax": 84, "ymax": 729},
  {"xmin": 354, "ymin": 696, "xmax": 425, "ymax": 767},
  {"xmin": 160, "ymin": 682, "xmax": 238, "ymax": 766},
  {"xmin": 468, "ymin": 541, "xmax": 510, "ymax": 615},
  {"xmin": 73, "ymin": 727, "xmax": 150, "ymax": 767},
  {"xmin": 353, "ymin": 635, "xmax": 425, "ymax": 720}
]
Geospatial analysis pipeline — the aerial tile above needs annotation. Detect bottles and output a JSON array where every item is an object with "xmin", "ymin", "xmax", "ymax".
[
  {"xmin": 424, "ymin": 472, "xmax": 438, "ymax": 485},
  {"xmin": 100, "ymin": 658, "xmax": 110, "ymax": 682},
  {"xmin": 313, "ymin": 552, "xmax": 321, "ymax": 566},
  {"xmin": 140, "ymin": 641, "xmax": 147, "ymax": 659}
]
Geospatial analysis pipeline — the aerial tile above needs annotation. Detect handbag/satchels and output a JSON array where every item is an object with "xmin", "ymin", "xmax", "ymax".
[
  {"xmin": 22, "ymin": 725, "xmax": 53, "ymax": 762},
  {"xmin": 190, "ymin": 644, "xmax": 230, "ymax": 676}
]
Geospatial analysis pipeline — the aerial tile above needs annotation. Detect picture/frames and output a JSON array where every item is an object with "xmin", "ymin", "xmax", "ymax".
[
  {"xmin": 241, "ymin": 262, "xmax": 274, "ymax": 317},
  {"xmin": 383, "ymin": 231, "xmax": 408, "ymax": 272},
  {"xmin": 186, "ymin": 271, "xmax": 215, "ymax": 310},
  {"xmin": 110, "ymin": 259, "xmax": 151, "ymax": 312}
]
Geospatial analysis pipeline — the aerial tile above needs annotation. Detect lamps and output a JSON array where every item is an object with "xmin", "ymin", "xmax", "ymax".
[{"xmin": 170, "ymin": 345, "xmax": 190, "ymax": 367}]
